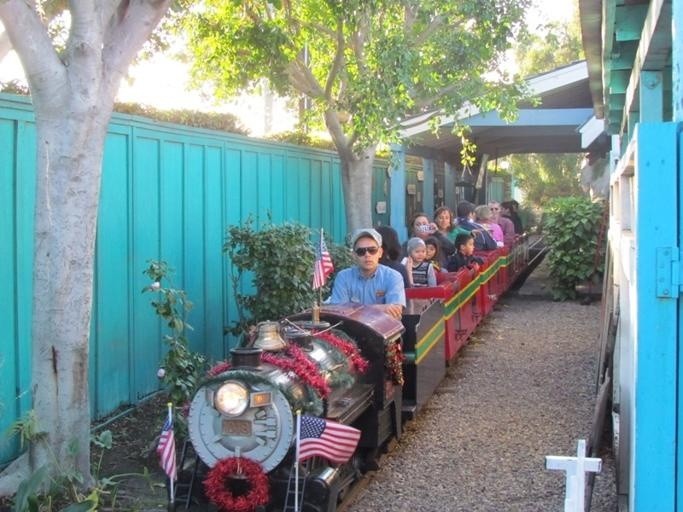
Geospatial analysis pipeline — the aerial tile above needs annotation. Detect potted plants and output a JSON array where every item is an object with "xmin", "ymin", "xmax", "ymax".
[{"xmin": 539, "ymin": 196, "xmax": 609, "ymax": 302}]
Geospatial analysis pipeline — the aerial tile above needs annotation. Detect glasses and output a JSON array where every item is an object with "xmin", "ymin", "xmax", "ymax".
[
  {"xmin": 356, "ymin": 246, "xmax": 381, "ymax": 257},
  {"xmin": 491, "ymin": 208, "xmax": 498, "ymax": 212}
]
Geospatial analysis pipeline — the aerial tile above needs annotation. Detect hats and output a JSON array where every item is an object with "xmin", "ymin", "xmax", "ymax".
[
  {"xmin": 352, "ymin": 228, "xmax": 383, "ymax": 248},
  {"xmin": 407, "ymin": 238, "xmax": 427, "ymax": 257}
]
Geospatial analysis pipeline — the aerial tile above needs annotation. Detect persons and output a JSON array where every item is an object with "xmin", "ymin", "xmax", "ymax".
[
  {"xmin": 432, "ymin": 199, "xmax": 523, "ymax": 271},
  {"xmin": 400, "ymin": 212, "xmax": 455, "ymax": 273},
  {"xmin": 375, "ymin": 225, "xmax": 413, "ymax": 288},
  {"xmin": 401, "ymin": 237, "xmax": 436, "ymax": 288},
  {"xmin": 423, "ymin": 237, "xmax": 441, "ymax": 271},
  {"xmin": 330, "ymin": 228, "xmax": 406, "ymax": 321}
]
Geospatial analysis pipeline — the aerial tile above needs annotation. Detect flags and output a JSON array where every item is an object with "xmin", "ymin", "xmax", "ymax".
[
  {"xmin": 298, "ymin": 414, "xmax": 362, "ymax": 463},
  {"xmin": 156, "ymin": 418, "xmax": 177, "ymax": 482},
  {"xmin": 312, "ymin": 234, "xmax": 333, "ymax": 291}
]
